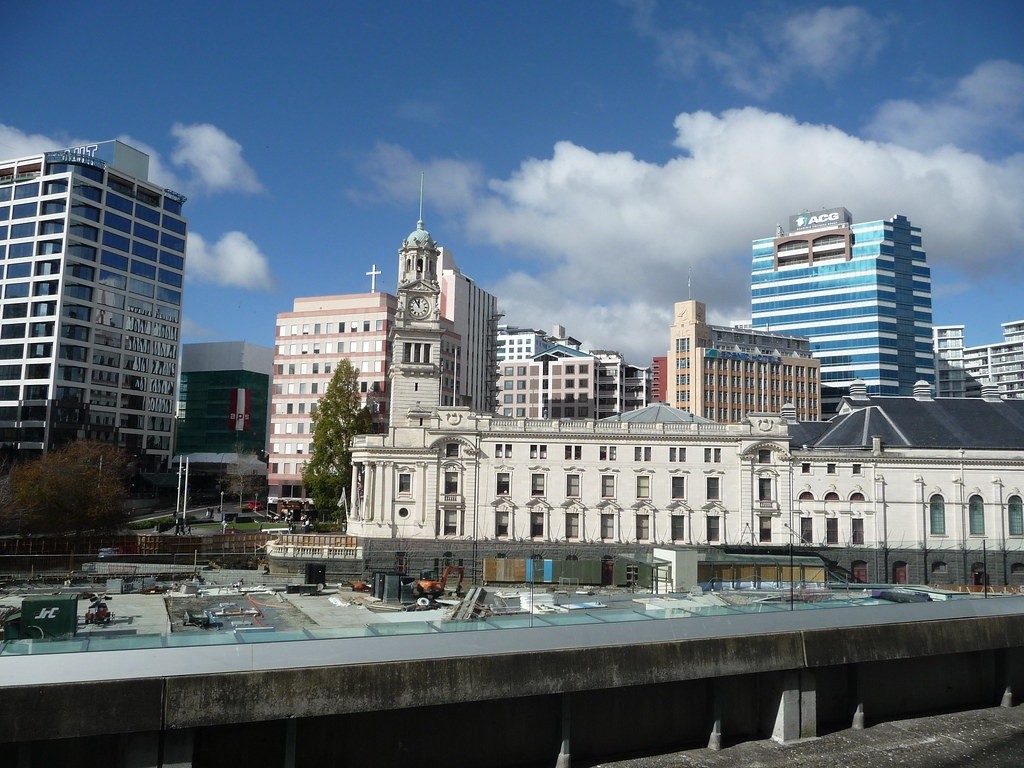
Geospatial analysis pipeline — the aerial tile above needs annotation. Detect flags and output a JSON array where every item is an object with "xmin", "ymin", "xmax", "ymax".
[{"xmin": 337, "ymin": 487, "xmax": 346, "ymax": 507}]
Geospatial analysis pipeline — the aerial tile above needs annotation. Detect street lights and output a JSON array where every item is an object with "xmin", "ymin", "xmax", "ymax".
[{"xmin": 221, "ymin": 491, "xmax": 225, "ymax": 512}]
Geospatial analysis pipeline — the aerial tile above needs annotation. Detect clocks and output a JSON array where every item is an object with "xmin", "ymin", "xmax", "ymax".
[{"xmin": 408, "ymin": 296, "xmax": 430, "ymax": 318}]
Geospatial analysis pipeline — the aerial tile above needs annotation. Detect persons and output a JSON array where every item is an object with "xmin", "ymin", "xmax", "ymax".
[
  {"xmin": 284, "ymin": 512, "xmax": 311, "ymax": 532},
  {"xmin": 205, "ymin": 508, "xmax": 212, "ymax": 518},
  {"xmin": 358, "ymin": 481, "xmax": 363, "ymax": 496},
  {"xmin": 174, "ymin": 523, "xmax": 193, "ymax": 536},
  {"xmin": 221, "ymin": 518, "xmax": 228, "ymax": 534},
  {"xmin": 355, "ymin": 492, "xmax": 359, "ymax": 507}
]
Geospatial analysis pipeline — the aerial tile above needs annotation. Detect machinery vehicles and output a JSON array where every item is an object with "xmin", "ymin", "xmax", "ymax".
[
  {"xmin": 84, "ymin": 596, "xmax": 113, "ymax": 624},
  {"xmin": 416, "ymin": 566, "xmax": 465, "ymax": 606}
]
